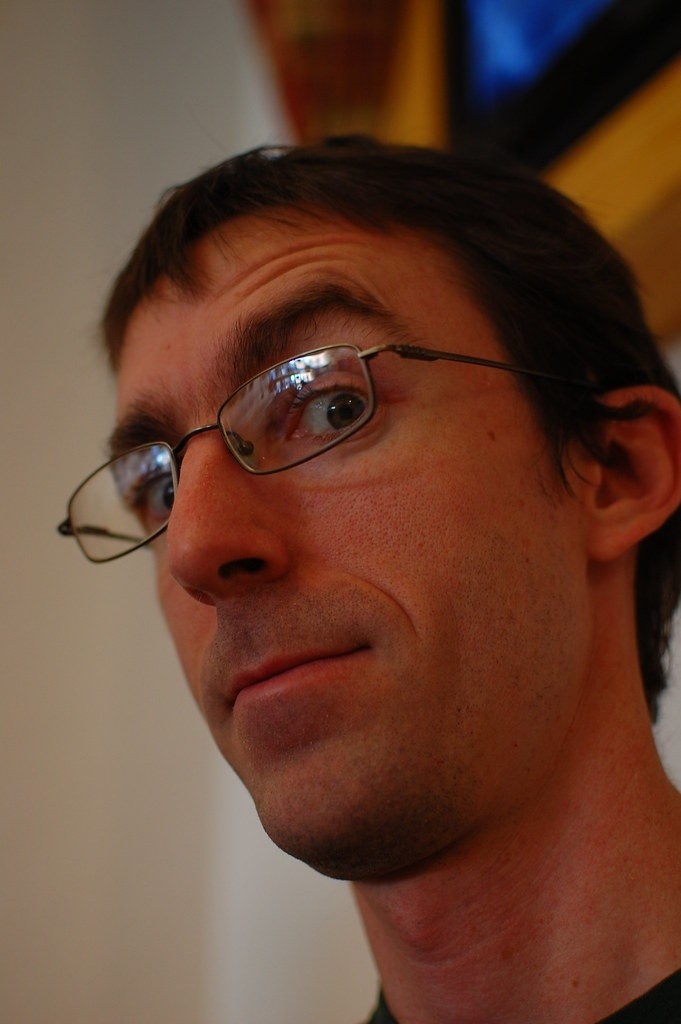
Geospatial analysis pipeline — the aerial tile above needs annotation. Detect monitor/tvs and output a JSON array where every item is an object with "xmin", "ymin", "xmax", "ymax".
[{"xmin": 440, "ymin": 0, "xmax": 681, "ymax": 180}]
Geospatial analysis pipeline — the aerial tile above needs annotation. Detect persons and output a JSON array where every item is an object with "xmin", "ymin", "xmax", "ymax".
[{"xmin": 54, "ymin": 134, "xmax": 680, "ymax": 1023}]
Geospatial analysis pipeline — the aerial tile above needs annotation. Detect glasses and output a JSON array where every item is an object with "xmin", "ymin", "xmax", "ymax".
[{"xmin": 56, "ymin": 342, "xmax": 600, "ymax": 567}]
